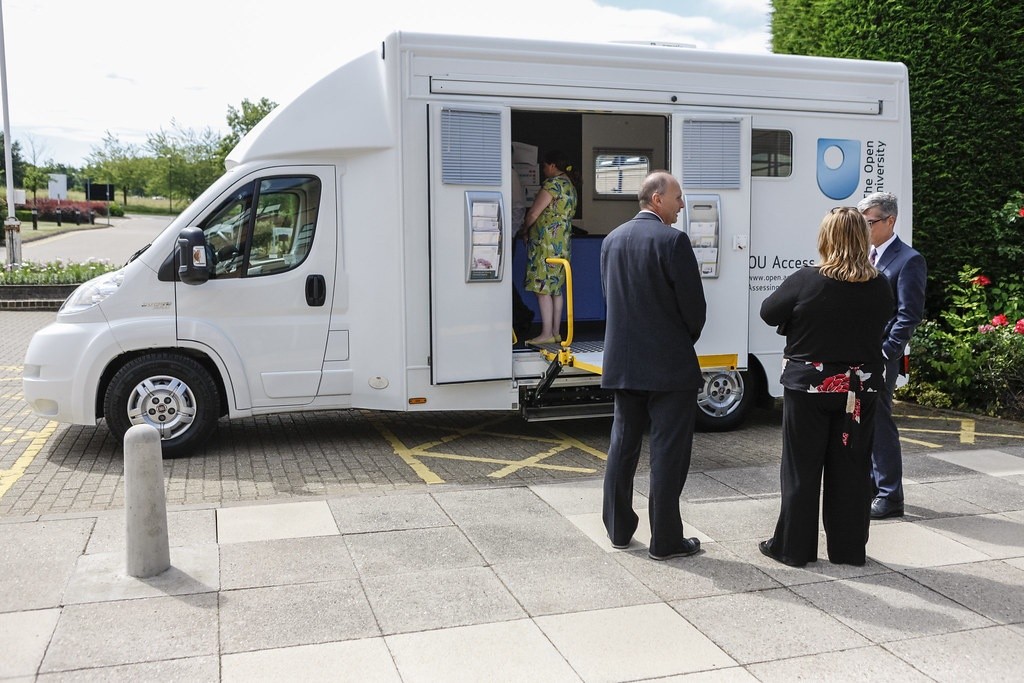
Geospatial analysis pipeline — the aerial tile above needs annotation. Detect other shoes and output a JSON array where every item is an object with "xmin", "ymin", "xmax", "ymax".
[
  {"xmin": 527, "ymin": 337, "xmax": 555, "ymax": 344},
  {"xmin": 759, "ymin": 540, "xmax": 806, "ymax": 567},
  {"xmin": 555, "ymin": 335, "xmax": 562, "ymax": 343}
]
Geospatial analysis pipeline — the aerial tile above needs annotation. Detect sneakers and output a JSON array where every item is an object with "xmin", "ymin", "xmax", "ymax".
[
  {"xmin": 870, "ymin": 497, "xmax": 904, "ymax": 519},
  {"xmin": 649, "ymin": 537, "xmax": 701, "ymax": 560},
  {"xmin": 608, "ymin": 532, "xmax": 632, "ymax": 548}
]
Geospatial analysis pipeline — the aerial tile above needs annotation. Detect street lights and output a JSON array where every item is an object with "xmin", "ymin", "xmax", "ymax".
[{"xmin": 166, "ymin": 154, "xmax": 173, "ymax": 214}]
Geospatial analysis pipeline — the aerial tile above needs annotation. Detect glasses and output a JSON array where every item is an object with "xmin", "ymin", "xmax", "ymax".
[
  {"xmin": 868, "ymin": 215, "xmax": 891, "ymax": 228},
  {"xmin": 830, "ymin": 206, "xmax": 858, "ymax": 215}
]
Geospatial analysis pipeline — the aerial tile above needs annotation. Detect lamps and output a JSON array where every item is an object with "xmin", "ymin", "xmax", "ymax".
[
  {"xmin": 91, "ymin": 210, "xmax": 94, "ymax": 215},
  {"xmin": 32, "ymin": 208, "xmax": 37, "ymax": 215},
  {"xmin": 76, "ymin": 209, "xmax": 80, "ymax": 215},
  {"xmin": 56, "ymin": 208, "xmax": 61, "ymax": 214}
]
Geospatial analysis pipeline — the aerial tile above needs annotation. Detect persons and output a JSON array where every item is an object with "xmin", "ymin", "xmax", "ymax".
[
  {"xmin": 759, "ymin": 204, "xmax": 895, "ymax": 563},
  {"xmin": 512, "ymin": 144, "xmax": 535, "ymax": 334},
  {"xmin": 524, "ymin": 151, "xmax": 579, "ymax": 344},
  {"xmin": 858, "ymin": 190, "xmax": 928, "ymax": 520},
  {"xmin": 600, "ymin": 170, "xmax": 707, "ymax": 560}
]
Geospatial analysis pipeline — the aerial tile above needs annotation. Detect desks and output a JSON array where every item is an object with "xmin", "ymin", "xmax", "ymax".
[{"xmin": 512, "ymin": 234, "xmax": 607, "ymax": 321}]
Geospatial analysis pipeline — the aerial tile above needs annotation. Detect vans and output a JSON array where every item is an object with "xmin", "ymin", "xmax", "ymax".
[{"xmin": 22, "ymin": 28, "xmax": 915, "ymax": 461}]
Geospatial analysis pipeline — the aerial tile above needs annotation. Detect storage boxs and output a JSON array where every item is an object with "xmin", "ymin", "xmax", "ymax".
[{"xmin": 512, "ymin": 164, "xmax": 542, "ymax": 208}]
